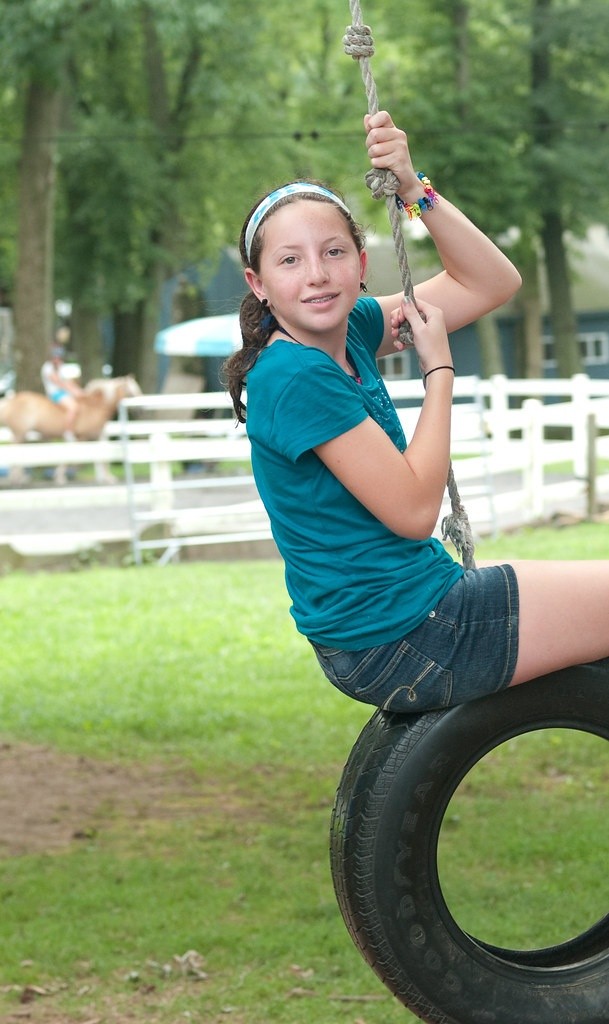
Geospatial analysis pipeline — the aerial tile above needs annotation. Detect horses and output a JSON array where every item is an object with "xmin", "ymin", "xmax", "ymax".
[{"xmin": 0, "ymin": 374, "xmax": 144, "ymax": 486}]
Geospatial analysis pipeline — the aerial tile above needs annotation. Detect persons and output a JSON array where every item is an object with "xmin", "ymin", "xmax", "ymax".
[
  {"xmin": 41, "ymin": 347, "xmax": 81, "ymax": 428},
  {"xmin": 222, "ymin": 111, "xmax": 608, "ymax": 712}
]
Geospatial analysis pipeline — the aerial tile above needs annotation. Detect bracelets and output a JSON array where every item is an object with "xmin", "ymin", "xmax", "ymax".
[
  {"xmin": 424, "ymin": 366, "xmax": 455, "ymax": 379},
  {"xmin": 396, "ymin": 171, "xmax": 439, "ymax": 221}
]
